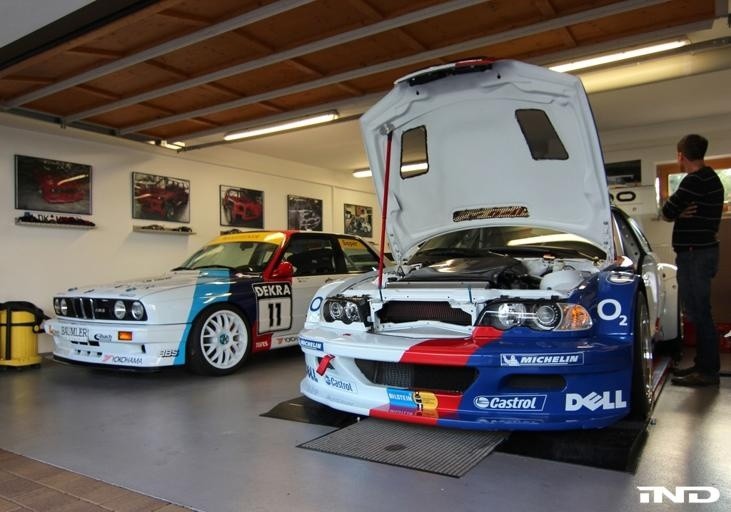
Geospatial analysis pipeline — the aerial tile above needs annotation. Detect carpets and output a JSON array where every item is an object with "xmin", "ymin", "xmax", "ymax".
[{"xmin": 259, "ymin": 396, "xmax": 649, "ymax": 477}]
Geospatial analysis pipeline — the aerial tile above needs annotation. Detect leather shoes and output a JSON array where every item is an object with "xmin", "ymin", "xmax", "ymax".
[
  {"xmin": 671, "ymin": 373, "xmax": 720, "ymax": 385},
  {"xmin": 671, "ymin": 367, "xmax": 693, "ymax": 376}
]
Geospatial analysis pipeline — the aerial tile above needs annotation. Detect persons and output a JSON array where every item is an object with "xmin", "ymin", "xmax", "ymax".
[{"xmin": 660, "ymin": 133, "xmax": 725, "ymax": 388}]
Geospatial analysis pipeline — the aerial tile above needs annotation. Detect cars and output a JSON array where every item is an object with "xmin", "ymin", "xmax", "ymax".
[
  {"xmin": 138, "ymin": 178, "xmax": 189, "ymax": 218},
  {"xmin": 289, "ymin": 198, "xmax": 320, "ymax": 230},
  {"xmin": 296, "ymin": 57, "xmax": 678, "ymax": 434},
  {"xmin": 345, "ymin": 209, "xmax": 371, "ymax": 232},
  {"xmin": 36, "ymin": 166, "xmax": 90, "ymax": 204},
  {"xmin": 44, "ymin": 230, "xmax": 408, "ymax": 377},
  {"xmin": 224, "ymin": 189, "xmax": 263, "ymax": 224}
]
